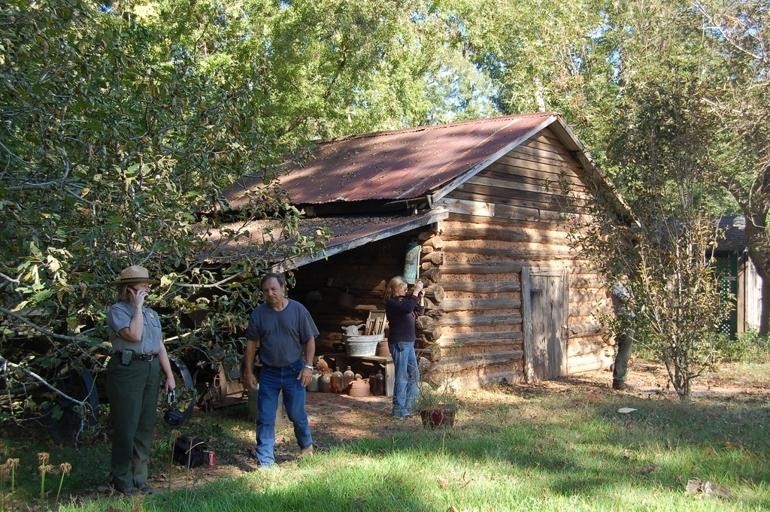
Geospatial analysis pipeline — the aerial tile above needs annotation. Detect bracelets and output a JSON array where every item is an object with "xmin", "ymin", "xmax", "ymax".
[{"xmin": 303, "ymin": 364, "xmax": 314, "ymax": 372}]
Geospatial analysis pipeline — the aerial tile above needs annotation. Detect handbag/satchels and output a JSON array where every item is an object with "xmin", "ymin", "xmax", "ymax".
[{"xmin": 172, "ymin": 435, "xmax": 203, "ymax": 468}]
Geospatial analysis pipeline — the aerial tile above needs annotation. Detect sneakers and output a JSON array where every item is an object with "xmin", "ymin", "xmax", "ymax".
[
  {"xmin": 301, "ymin": 444, "xmax": 314, "ymax": 457},
  {"xmin": 116, "ymin": 485, "xmax": 156, "ymax": 496}
]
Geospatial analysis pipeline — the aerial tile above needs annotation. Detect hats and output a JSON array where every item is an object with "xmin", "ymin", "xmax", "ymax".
[{"xmin": 112, "ymin": 265, "xmax": 160, "ymax": 284}]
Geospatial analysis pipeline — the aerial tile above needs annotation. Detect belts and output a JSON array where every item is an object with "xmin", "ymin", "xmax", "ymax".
[{"xmin": 116, "ymin": 351, "xmax": 158, "ymax": 361}]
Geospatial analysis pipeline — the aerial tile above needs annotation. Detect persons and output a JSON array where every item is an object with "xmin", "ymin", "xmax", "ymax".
[
  {"xmin": 244, "ymin": 273, "xmax": 320, "ymax": 469},
  {"xmin": 381, "ymin": 274, "xmax": 427, "ymax": 418},
  {"xmin": 106, "ymin": 265, "xmax": 176, "ymax": 497},
  {"xmin": 610, "ymin": 272, "xmax": 638, "ymax": 391}
]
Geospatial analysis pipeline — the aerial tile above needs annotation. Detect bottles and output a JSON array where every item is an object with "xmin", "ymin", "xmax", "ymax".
[
  {"xmin": 376, "ymin": 370, "xmax": 385, "ymax": 394},
  {"xmin": 331, "ymin": 366, "xmax": 355, "ymax": 393}
]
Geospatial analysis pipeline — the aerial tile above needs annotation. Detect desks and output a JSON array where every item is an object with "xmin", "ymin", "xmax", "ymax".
[{"xmin": 331, "ymin": 352, "xmax": 395, "ymax": 397}]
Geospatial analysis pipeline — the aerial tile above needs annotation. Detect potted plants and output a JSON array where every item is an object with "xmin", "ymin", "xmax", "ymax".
[{"xmin": 410, "ymin": 338, "xmax": 459, "ymax": 431}]
